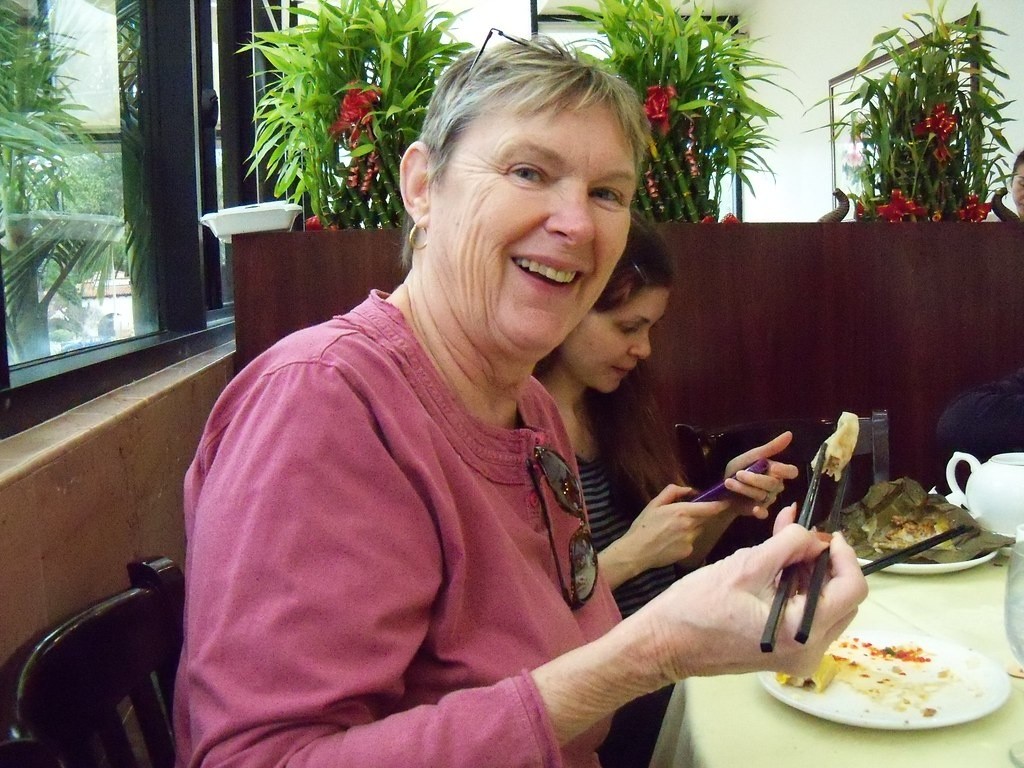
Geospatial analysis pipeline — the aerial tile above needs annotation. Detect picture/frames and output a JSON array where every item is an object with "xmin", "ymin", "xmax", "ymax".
[{"xmin": 827, "ymin": 13, "xmax": 980, "ymax": 222}]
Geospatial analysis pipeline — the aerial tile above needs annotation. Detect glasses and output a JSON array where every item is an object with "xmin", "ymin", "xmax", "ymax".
[
  {"xmin": 469, "ymin": 29, "xmax": 624, "ymax": 94},
  {"xmin": 525, "ymin": 445, "xmax": 599, "ymax": 612}
]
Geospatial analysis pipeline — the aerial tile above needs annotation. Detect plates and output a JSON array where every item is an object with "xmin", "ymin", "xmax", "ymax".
[
  {"xmin": 758, "ymin": 632, "xmax": 1011, "ymax": 729},
  {"xmin": 856, "ymin": 550, "xmax": 997, "ymax": 575}
]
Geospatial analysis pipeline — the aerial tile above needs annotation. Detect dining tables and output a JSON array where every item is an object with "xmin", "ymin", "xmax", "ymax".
[{"xmin": 684, "ymin": 555, "xmax": 1024, "ymax": 768}]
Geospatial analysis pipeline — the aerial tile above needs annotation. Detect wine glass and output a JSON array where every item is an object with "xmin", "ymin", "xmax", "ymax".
[{"xmin": 1004, "ymin": 542, "xmax": 1024, "ymax": 768}]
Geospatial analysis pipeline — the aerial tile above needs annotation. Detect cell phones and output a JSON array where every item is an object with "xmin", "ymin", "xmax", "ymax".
[{"xmin": 691, "ymin": 457, "xmax": 770, "ymax": 502}]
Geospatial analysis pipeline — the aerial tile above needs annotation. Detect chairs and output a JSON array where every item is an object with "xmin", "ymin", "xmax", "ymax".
[
  {"xmin": 0, "ymin": 553, "xmax": 185, "ymax": 768},
  {"xmin": 674, "ymin": 407, "xmax": 892, "ymax": 559}
]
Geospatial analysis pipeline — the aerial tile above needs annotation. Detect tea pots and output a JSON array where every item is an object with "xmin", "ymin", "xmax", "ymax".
[{"xmin": 945, "ymin": 452, "xmax": 1024, "ymax": 534}]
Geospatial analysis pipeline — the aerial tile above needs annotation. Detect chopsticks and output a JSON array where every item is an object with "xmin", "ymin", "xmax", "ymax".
[
  {"xmin": 861, "ymin": 525, "xmax": 975, "ymax": 576},
  {"xmin": 761, "ymin": 443, "xmax": 848, "ymax": 652}
]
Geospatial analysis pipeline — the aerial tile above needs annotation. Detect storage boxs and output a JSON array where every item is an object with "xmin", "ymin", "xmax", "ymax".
[
  {"xmin": 4, "ymin": 208, "xmax": 126, "ymax": 243},
  {"xmin": 199, "ymin": 199, "xmax": 302, "ymax": 244}
]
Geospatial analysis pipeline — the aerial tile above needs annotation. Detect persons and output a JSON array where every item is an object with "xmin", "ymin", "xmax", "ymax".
[
  {"xmin": 532, "ymin": 206, "xmax": 800, "ymax": 768},
  {"xmin": 170, "ymin": 36, "xmax": 867, "ymax": 768},
  {"xmin": 1009, "ymin": 148, "xmax": 1024, "ymax": 223},
  {"xmin": 935, "ymin": 368, "xmax": 1024, "ymax": 468}
]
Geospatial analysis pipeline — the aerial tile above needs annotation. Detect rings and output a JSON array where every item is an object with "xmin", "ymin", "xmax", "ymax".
[{"xmin": 761, "ymin": 492, "xmax": 770, "ymax": 504}]
